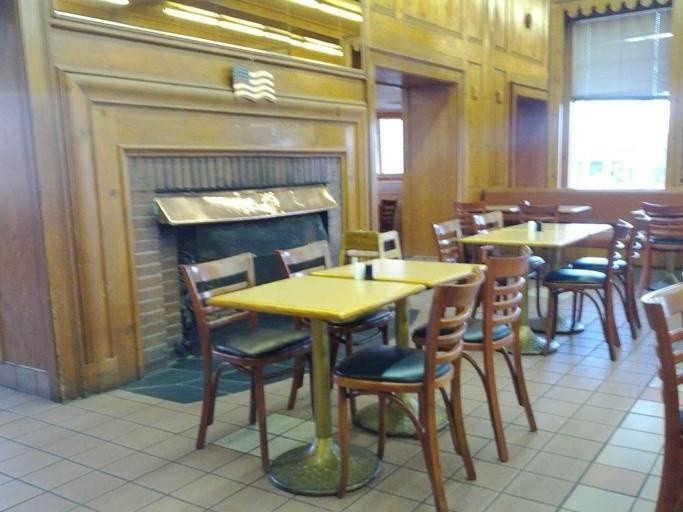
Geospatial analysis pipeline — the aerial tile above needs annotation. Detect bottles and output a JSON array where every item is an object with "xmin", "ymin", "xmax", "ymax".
[
  {"xmin": 528, "ymin": 220, "xmax": 543, "ymax": 232},
  {"xmin": 353, "ymin": 263, "xmax": 374, "ymax": 280},
  {"xmin": 521, "ymin": 200, "xmax": 531, "ymax": 206}
]
